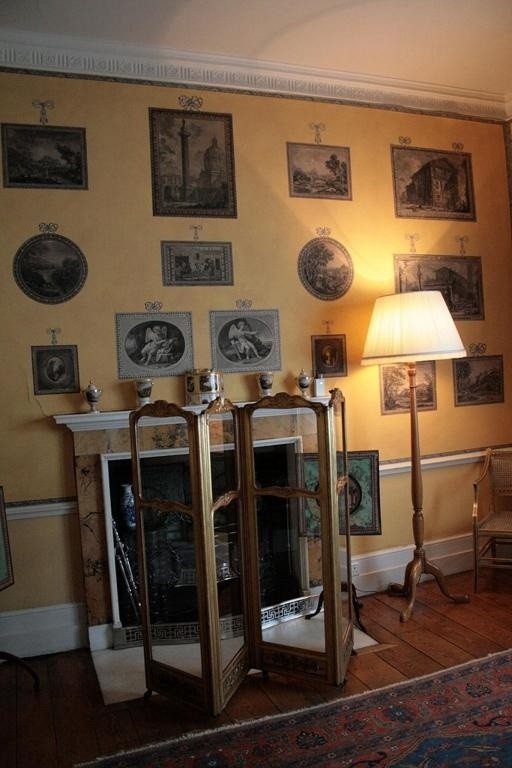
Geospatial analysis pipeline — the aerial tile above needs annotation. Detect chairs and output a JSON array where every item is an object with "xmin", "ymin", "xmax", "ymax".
[{"xmin": 473, "ymin": 447, "xmax": 512, "ymax": 593}]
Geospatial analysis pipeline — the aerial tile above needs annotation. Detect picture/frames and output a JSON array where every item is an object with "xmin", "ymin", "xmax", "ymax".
[
  {"xmin": 391, "ymin": 145, "xmax": 476, "ymax": 220},
  {"xmin": 298, "ymin": 450, "xmax": 382, "ymax": 534},
  {"xmin": 149, "ymin": 108, "xmax": 238, "ymax": 218},
  {"xmin": 1, "ymin": 122, "xmax": 89, "ymax": 189},
  {"xmin": 115, "ymin": 310, "xmax": 195, "ymax": 379},
  {"xmin": 453, "ymin": 355, "xmax": 505, "ymax": 407},
  {"xmin": 0, "ymin": 485, "xmax": 14, "ymax": 593},
  {"xmin": 297, "ymin": 237, "xmax": 353, "ymax": 300},
  {"xmin": 12, "ymin": 235, "xmax": 88, "ymax": 304},
  {"xmin": 209, "ymin": 308, "xmax": 282, "ymax": 372},
  {"xmin": 288, "ymin": 141, "xmax": 352, "ymax": 202},
  {"xmin": 393, "ymin": 253, "xmax": 484, "ymax": 321},
  {"xmin": 380, "ymin": 359, "xmax": 437, "ymax": 415},
  {"xmin": 31, "ymin": 346, "xmax": 82, "ymax": 396},
  {"xmin": 160, "ymin": 240, "xmax": 234, "ymax": 285},
  {"xmin": 312, "ymin": 334, "xmax": 347, "ymax": 377}
]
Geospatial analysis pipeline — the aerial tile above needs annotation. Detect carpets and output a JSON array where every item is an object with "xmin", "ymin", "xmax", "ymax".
[{"xmin": 57, "ymin": 644, "xmax": 512, "ymax": 763}]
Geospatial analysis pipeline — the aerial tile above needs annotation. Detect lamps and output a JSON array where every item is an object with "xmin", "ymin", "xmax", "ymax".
[{"xmin": 361, "ymin": 290, "xmax": 469, "ymax": 624}]
